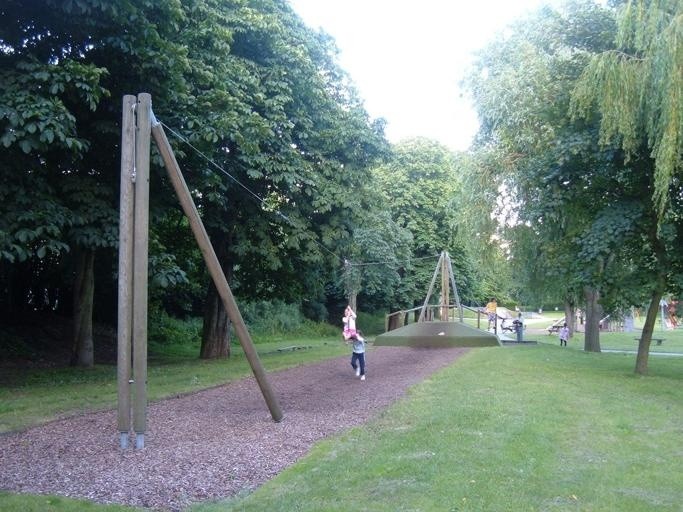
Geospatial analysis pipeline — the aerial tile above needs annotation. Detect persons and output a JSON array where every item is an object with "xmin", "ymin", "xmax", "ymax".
[
  {"xmin": 340, "ymin": 304, "xmax": 358, "ymax": 344},
  {"xmin": 558, "ymin": 323, "xmax": 569, "ymax": 347},
  {"xmin": 484, "ymin": 297, "xmax": 497, "ymax": 332},
  {"xmin": 511, "ymin": 312, "xmax": 523, "ymax": 341},
  {"xmin": 344, "ymin": 328, "xmax": 366, "ymax": 382}
]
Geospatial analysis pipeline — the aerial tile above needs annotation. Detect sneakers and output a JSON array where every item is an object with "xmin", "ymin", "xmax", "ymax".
[
  {"xmin": 354, "ymin": 366, "xmax": 361, "ymax": 378},
  {"xmin": 360, "ymin": 374, "xmax": 366, "ymax": 381}
]
